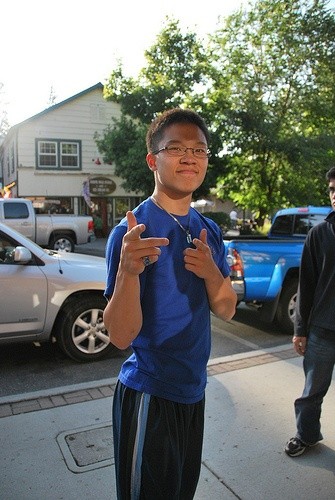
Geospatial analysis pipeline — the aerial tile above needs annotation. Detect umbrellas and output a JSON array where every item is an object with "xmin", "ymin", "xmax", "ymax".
[{"xmin": 190, "ymin": 199, "xmax": 215, "ymax": 213}]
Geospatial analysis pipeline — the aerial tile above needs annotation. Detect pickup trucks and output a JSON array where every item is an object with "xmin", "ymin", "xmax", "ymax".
[
  {"xmin": 223, "ymin": 206, "xmax": 334, "ymax": 332},
  {"xmin": 0, "ymin": 194, "xmax": 96, "ymax": 254}
]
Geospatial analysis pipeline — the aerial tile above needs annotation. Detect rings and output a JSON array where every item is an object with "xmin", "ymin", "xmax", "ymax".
[{"xmin": 142, "ymin": 256, "xmax": 150, "ymax": 266}]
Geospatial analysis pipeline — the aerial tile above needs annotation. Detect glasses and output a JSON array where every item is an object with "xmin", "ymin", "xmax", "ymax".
[{"xmin": 152, "ymin": 145, "xmax": 212, "ymax": 158}]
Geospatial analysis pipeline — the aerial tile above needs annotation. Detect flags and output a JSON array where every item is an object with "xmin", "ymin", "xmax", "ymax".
[{"xmin": 81, "ymin": 178, "xmax": 92, "ymax": 208}]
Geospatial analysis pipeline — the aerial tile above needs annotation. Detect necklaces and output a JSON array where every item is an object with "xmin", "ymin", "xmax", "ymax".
[{"xmin": 151, "ymin": 194, "xmax": 193, "ymax": 243}]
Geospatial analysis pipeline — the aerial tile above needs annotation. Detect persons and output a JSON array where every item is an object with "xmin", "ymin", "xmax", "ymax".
[
  {"xmin": 229, "ymin": 209, "xmax": 238, "ymax": 230},
  {"xmin": 91, "ymin": 210, "xmax": 108, "ymax": 239},
  {"xmin": 283, "ymin": 166, "xmax": 335, "ymax": 456},
  {"xmin": 103, "ymin": 108, "xmax": 238, "ymax": 500}
]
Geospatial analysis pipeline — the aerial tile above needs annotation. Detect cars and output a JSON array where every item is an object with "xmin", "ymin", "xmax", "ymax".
[{"xmin": 0, "ymin": 220, "xmax": 115, "ymax": 362}]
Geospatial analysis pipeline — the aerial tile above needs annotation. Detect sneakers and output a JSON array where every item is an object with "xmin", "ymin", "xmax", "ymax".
[{"xmin": 285, "ymin": 437, "xmax": 313, "ymax": 457}]
